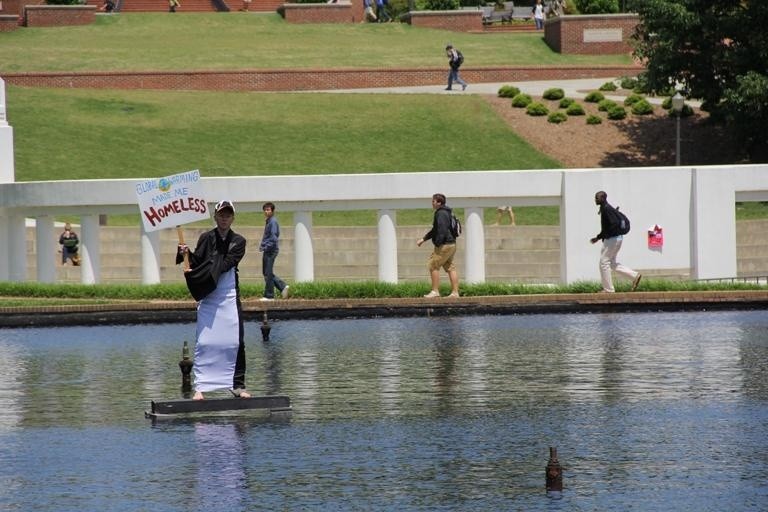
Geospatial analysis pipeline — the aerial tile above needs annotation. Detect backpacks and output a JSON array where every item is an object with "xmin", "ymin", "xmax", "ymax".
[
  {"xmin": 449, "ymin": 213, "xmax": 462, "ymax": 237},
  {"xmin": 605, "ymin": 204, "xmax": 630, "ymax": 235}
]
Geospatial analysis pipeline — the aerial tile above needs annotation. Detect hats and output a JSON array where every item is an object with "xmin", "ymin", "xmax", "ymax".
[{"xmin": 215, "ymin": 200, "xmax": 235, "ymax": 215}]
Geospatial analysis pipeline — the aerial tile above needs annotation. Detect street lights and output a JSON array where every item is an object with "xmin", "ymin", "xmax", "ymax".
[{"xmin": 673, "ymin": 92, "xmax": 685, "ymax": 166}]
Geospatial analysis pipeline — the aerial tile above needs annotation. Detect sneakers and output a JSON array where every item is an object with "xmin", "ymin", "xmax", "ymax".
[
  {"xmin": 281, "ymin": 285, "xmax": 289, "ymax": 298},
  {"xmin": 598, "ymin": 288, "xmax": 615, "ymax": 293},
  {"xmin": 632, "ymin": 274, "xmax": 641, "ymax": 292},
  {"xmin": 423, "ymin": 289, "xmax": 440, "ymax": 298},
  {"xmin": 258, "ymin": 297, "xmax": 274, "ymax": 301},
  {"xmin": 446, "ymin": 291, "xmax": 459, "ymax": 298}
]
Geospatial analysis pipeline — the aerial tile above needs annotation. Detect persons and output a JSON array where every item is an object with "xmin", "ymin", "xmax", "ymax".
[
  {"xmin": 416, "ymin": 194, "xmax": 461, "ymax": 299},
  {"xmin": 362, "ymin": 0, "xmax": 393, "ymax": 24},
  {"xmin": 99, "ymin": 0, "xmax": 119, "ymax": 14},
  {"xmin": 589, "ymin": 190, "xmax": 641, "ymax": 293},
  {"xmin": 492, "ymin": 206, "xmax": 516, "ymax": 226},
  {"xmin": 258, "ymin": 202, "xmax": 290, "ymax": 302},
  {"xmin": 168, "ymin": 1, "xmax": 181, "ymax": 13},
  {"xmin": 58, "ymin": 222, "xmax": 81, "ymax": 265},
  {"xmin": 532, "ymin": 0, "xmax": 545, "ymax": 30},
  {"xmin": 176, "ymin": 198, "xmax": 252, "ymax": 401},
  {"xmin": 443, "ymin": 45, "xmax": 467, "ymax": 91}
]
